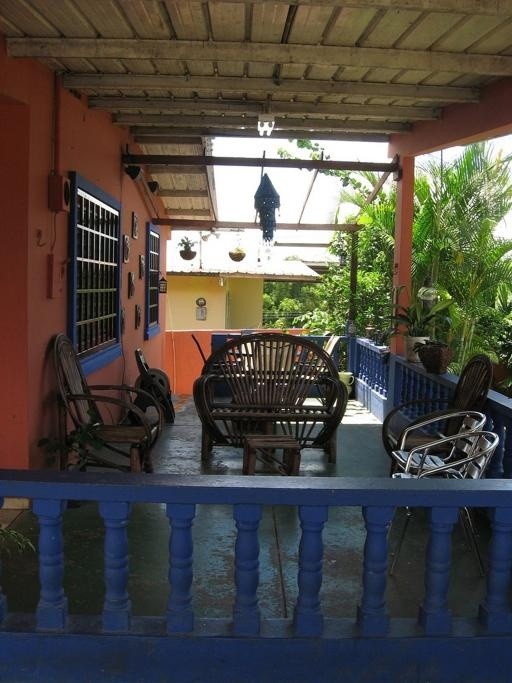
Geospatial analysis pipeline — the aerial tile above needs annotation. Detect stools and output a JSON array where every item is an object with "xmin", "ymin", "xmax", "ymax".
[{"xmin": 242, "ymin": 436, "xmax": 299, "ymax": 472}]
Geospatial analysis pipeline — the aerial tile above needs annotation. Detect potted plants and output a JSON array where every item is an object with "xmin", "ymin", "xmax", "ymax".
[
  {"xmin": 365, "ymin": 312, "xmax": 374, "ymax": 338},
  {"xmin": 178, "ymin": 238, "xmax": 196, "ymax": 260},
  {"xmin": 230, "ymin": 248, "xmax": 244, "ymax": 263},
  {"xmin": 388, "ymin": 293, "xmax": 454, "ymax": 364},
  {"xmin": 413, "ymin": 340, "xmax": 451, "ymax": 372}
]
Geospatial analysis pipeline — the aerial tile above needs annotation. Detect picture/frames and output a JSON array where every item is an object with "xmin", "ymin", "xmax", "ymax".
[
  {"xmin": 136, "ymin": 305, "xmax": 141, "ymax": 329},
  {"xmin": 141, "ymin": 256, "xmax": 145, "ymax": 279},
  {"xmin": 123, "ymin": 236, "xmax": 130, "ymax": 264},
  {"xmin": 129, "ymin": 272, "xmax": 134, "ymax": 299},
  {"xmin": 131, "ymin": 214, "xmax": 139, "ymax": 240}
]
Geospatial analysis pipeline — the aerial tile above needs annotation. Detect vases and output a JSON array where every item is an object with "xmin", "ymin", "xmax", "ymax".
[{"xmin": 339, "ymin": 372, "xmax": 355, "ymax": 398}]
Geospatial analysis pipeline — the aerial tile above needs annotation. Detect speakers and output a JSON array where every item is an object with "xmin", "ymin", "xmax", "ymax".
[
  {"xmin": 128, "ymin": 165, "xmax": 144, "ymax": 181},
  {"xmin": 152, "ymin": 181, "xmax": 159, "ymax": 194}
]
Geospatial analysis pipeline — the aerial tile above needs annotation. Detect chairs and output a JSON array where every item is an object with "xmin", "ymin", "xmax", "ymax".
[
  {"xmin": 53, "ymin": 333, "xmax": 164, "ymax": 475},
  {"xmin": 383, "ymin": 353, "xmax": 494, "ymax": 471},
  {"xmin": 194, "ymin": 333, "xmax": 348, "ymax": 463},
  {"xmin": 389, "ymin": 431, "xmax": 500, "ymax": 572},
  {"xmin": 393, "ymin": 410, "xmax": 486, "ymax": 470}
]
